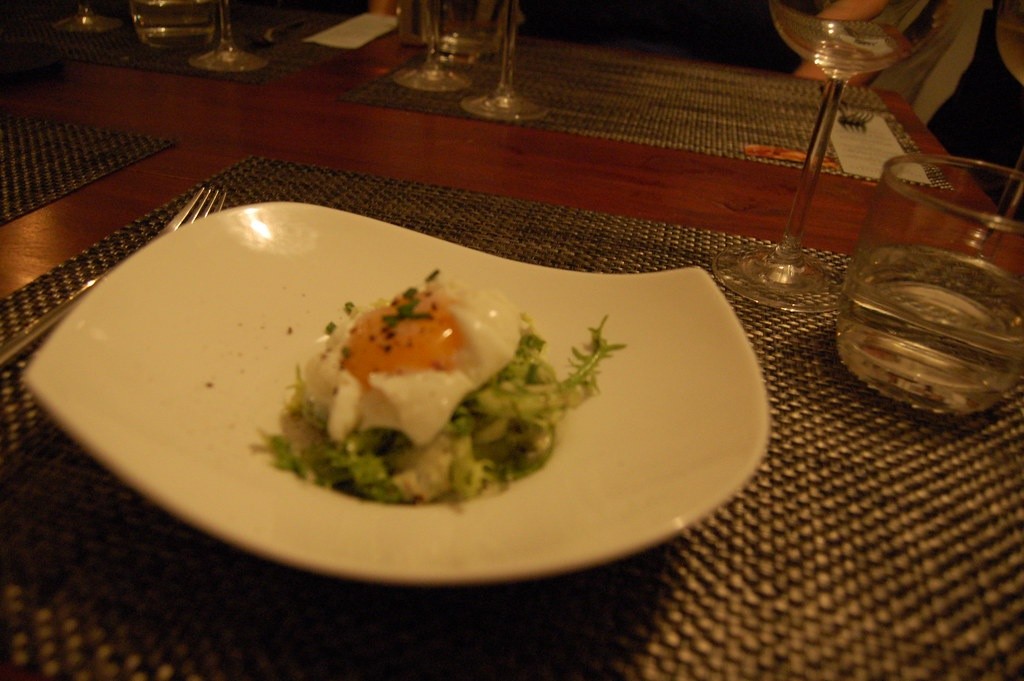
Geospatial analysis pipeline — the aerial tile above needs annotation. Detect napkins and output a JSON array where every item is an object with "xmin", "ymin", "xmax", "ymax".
[
  {"xmin": 828, "ymin": 110, "xmax": 935, "ymax": 183},
  {"xmin": 301, "ymin": 12, "xmax": 397, "ymax": 51}
]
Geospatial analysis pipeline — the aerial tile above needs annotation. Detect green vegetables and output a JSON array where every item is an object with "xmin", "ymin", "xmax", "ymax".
[{"xmin": 253, "ymin": 315, "xmax": 628, "ymax": 504}]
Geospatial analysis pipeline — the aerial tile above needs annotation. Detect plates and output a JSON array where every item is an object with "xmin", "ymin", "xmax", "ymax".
[{"xmin": 25, "ymin": 200, "xmax": 769, "ymax": 585}]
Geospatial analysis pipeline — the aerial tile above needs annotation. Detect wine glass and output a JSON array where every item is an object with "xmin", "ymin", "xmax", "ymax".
[
  {"xmin": 393, "ymin": 0, "xmax": 552, "ymax": 124},
  {"xmin": 714, "ymin": 0, "xmax": 938, "ymax": 313},
  {"xmin": 188, "ymin": 0, "xmax": 270, "ymax": 72},
  {"xmin": 51, "ymin": 0, "xmax": 124, "ymax": 33}
]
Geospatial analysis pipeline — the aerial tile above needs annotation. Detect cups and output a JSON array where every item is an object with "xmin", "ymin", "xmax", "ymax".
[
  {"xmin": 439, "ymin": 0, "xmax": 508, "ymax": 54},
  {"xmin": 838, "ymin": 153, "xmax": 1024, "ymax": 419},
  {"xmin": 129, "ymin": 0, "xmax": 217, "ymax": 49}
]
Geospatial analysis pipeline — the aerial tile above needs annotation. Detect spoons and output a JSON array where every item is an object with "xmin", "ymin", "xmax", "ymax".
[{"xmin": 0, "ymin": 186, "xmax": 227, "ymax": 367}]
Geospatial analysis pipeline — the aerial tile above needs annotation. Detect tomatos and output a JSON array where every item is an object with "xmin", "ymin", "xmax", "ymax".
[{"xmin": 339, "ymin": 277, "xmax": 472, "ymax": 386}]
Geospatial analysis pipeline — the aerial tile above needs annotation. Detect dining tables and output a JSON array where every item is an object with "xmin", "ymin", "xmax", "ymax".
[{"xmin": 0, "ymin": 0, "xmax": 1024, "ymax": 680}]
